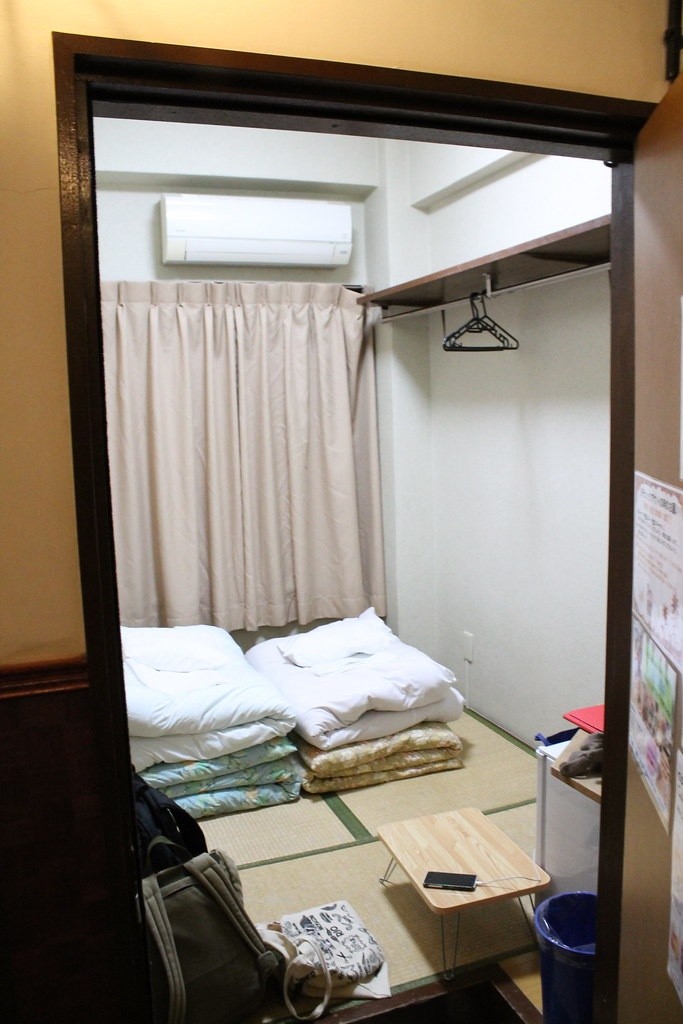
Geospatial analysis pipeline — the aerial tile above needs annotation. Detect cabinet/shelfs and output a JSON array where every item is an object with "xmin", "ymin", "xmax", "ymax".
[{"xmin": 531, "ymin": 742, "xmax": 603, "ymax": 911}]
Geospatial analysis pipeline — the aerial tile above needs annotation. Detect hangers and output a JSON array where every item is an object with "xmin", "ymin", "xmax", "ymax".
[{"xmin": 442, "ymin": 290, "xmax": 520, "ymax": 351}]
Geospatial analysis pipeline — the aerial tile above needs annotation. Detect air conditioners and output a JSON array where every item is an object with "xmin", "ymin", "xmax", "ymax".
[{"xmin": 162, "ymin": 192, "xmax": 353, "ymax": 270}]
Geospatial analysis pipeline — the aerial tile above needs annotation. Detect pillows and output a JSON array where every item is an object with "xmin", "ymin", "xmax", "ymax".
[{"xmin": 280, "ymin": 616, "xmax": 399, "ymax": 670}]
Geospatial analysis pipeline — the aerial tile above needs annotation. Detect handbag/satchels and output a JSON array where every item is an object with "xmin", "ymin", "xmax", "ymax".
[{"xmin": 253, "ymin": 899, "xmax": 392, "ymax": 1021}]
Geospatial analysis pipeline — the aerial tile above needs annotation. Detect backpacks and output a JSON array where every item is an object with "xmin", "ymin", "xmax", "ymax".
[
  {"xmin": 130, "ymin": 763, "xmax": 209, "ymax": 876},
  {"xmin": 138, "ymin": 836, "xmax": 280, "ymax": 1023}
]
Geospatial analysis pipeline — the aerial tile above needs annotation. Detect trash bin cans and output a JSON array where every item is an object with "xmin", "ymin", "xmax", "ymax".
[{"xmin": 534, "ymin": 890, "xmax": 597, "ymax": 1024}]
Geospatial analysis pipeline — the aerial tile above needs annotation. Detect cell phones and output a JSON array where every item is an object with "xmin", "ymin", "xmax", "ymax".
[{"xmin": 423, "ymin": 871, "xmax": 477, "ymax": 892}]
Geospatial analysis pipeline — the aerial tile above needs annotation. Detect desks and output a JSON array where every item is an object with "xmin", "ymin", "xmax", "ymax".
[{"xmin": 378, "ymin": 806, "xmax": 551, "ymax": 982}]
